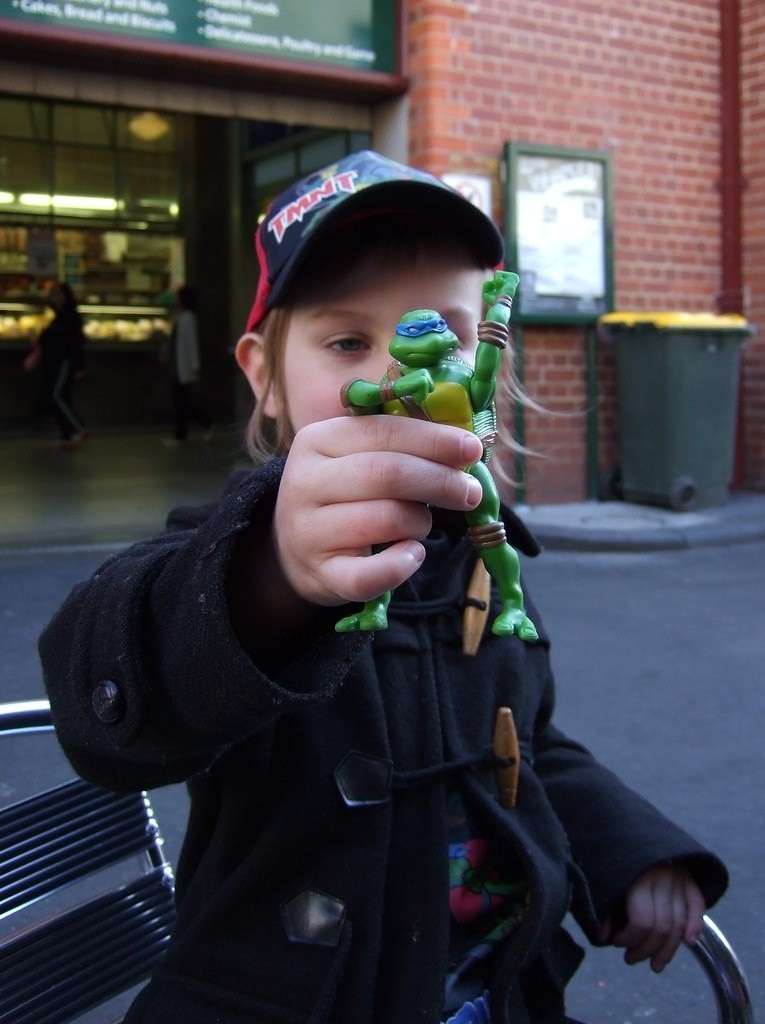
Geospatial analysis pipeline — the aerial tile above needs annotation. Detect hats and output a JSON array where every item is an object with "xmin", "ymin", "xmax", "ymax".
[{"xmin": 244, "ymin": 148, "xmax": 505, "ymax": 333}]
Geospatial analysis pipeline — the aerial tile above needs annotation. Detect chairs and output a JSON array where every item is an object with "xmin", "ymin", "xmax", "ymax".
[{"xmin": 0, "ymin": 639, "xmax": 753, "ymax": 1024}]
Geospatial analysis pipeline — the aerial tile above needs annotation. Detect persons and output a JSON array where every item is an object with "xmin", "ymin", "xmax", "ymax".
[
  {"xmin": 39, "ymin": 150, "xmax": 729, "ymax": 1024},
  {"xmin": 24, "ymin": 281, "xmax": 87, "ymax": 450},
  {"xmin": 164, "ymin": 286, "xmax": 214, "ymax": 444}
]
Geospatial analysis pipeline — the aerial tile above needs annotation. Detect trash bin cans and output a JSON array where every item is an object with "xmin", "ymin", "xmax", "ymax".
[{"xmin": 584, "ymin": 312, "xmax": 754, "ymax": 515}]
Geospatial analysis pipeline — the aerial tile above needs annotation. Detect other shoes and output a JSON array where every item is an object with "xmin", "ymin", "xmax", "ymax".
[{"xmin": 65, "ymin": 430, "xmax": 89, "ymax": 448}]
